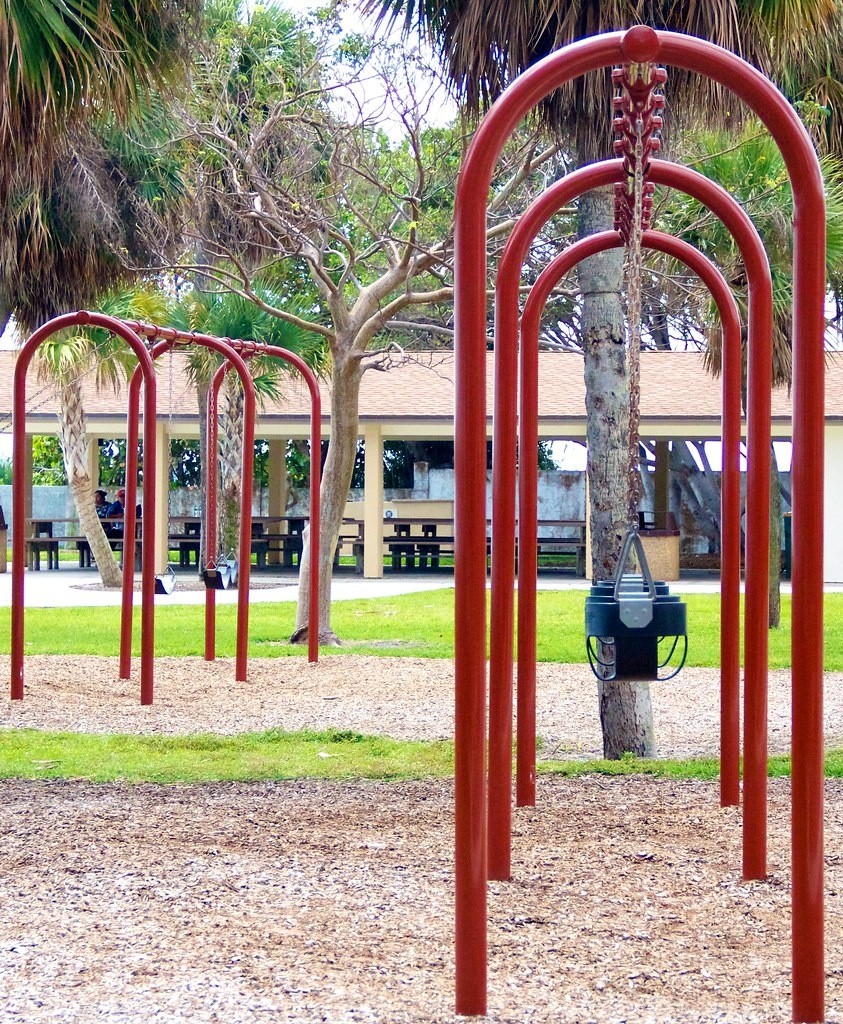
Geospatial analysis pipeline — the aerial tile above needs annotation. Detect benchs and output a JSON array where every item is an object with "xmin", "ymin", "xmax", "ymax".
[
  {"xmin": 516, "ymin": 537, "xmax": 584, "ymax": 547},
  {"xmin": 53, "ymin": 536, "xmax": 129, "ymax": 569},
  {"xmin": 384, "ymin": 537, "xmax": 492, "ymax": 546},
  {"xmin": 169, "ymin": 538, "xmax": 268, "ymax": 544},
  {"xmin": 25, "ymin": 537, "xmax": 139, "ymax": 571}
]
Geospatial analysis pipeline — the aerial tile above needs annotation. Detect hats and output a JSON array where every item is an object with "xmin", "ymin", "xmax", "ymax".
[{"xmin": 118, "ymin": 490, "xmax": 125, "ymax": 497}]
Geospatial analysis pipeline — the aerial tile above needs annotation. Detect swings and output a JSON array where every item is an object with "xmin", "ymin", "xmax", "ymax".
[
  {"xmin": 203, "ymin": 348, "xmax": 239, "ymax": 591},
  {"xmin": 146, "ymin": 336, "xmax": 178, "ymax": 596},
  {"xmin": 582, "ymin": 115, "xmax": 690, "ymax": 684}
]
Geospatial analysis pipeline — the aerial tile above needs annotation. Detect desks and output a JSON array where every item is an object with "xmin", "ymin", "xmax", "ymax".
[
  {"xmin": 26, "ymin": 517, "xmax": 143, "ymax": 571},
  {"xmin": 168, "ymin": 518, "xmax": 308, "ymax": 570},
  {"xmin": 383, "ymin": 517, "xmax": 491, "ymax": 570},
  {"xmin": 515, "ymin": 519, "xmax": 585, "ymax": 574}
]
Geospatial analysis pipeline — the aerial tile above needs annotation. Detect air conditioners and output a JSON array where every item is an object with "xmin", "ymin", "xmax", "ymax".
[{"xmin": 383, "ymin": 508, "xmax": 397, "ymax": 519}]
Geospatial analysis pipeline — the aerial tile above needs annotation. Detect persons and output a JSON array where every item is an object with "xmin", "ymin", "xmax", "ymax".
[{"xmin": 94, "ymin": 489, "xmax": 125, "ymax": 550}]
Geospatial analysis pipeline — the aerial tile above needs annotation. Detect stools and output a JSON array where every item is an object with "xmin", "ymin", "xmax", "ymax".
[{"xmin": 258, "ymin": 534, "xmax": 301, "ymax": 570}]
